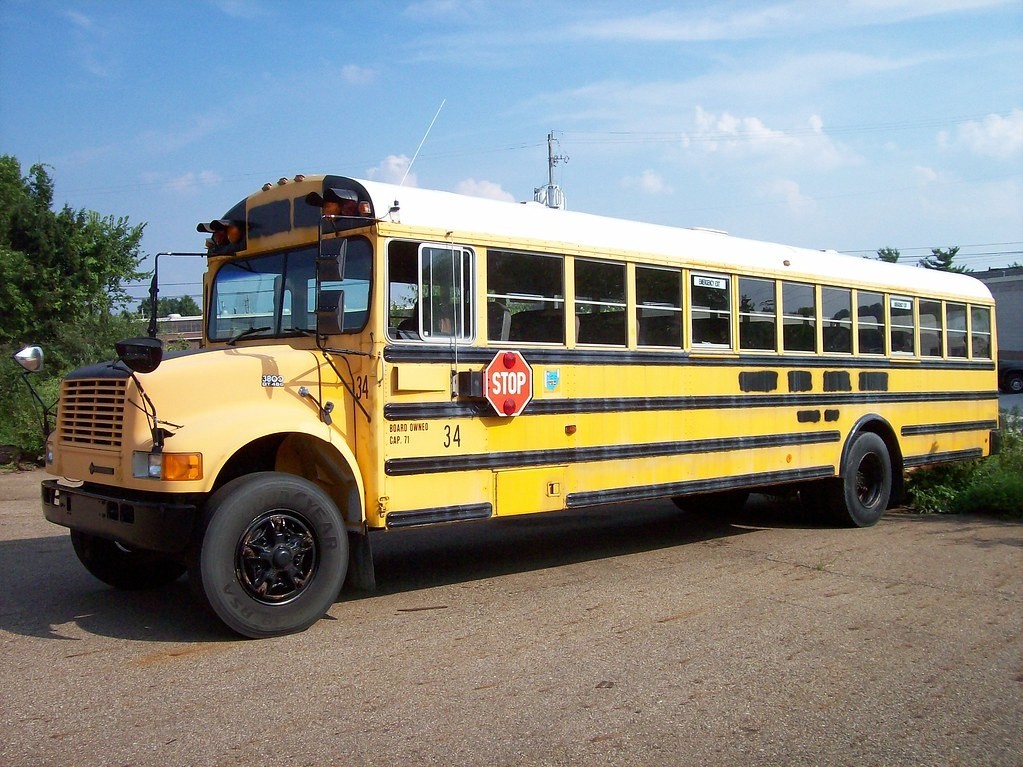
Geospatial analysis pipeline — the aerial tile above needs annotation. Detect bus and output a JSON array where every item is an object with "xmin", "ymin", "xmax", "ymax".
[{"xmin": 13, "ymin": 174, "xmax": 1003, "ymax": 638}]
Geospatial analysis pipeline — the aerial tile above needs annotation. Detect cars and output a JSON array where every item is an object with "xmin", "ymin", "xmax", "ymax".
[{"xmin": 998, "ymin": 360, "xmax": 1023, "ymax": 393}]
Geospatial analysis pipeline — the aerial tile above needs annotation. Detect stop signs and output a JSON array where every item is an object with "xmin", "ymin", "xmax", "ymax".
[{"xmin": 486, "ymin": 350, "xmax": 532, "ymax": 417}]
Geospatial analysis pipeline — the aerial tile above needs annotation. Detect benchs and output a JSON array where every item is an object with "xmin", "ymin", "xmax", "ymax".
[{"xmin": 482, "ymin": 300, "xmax": 990, "ymax": 360}]
